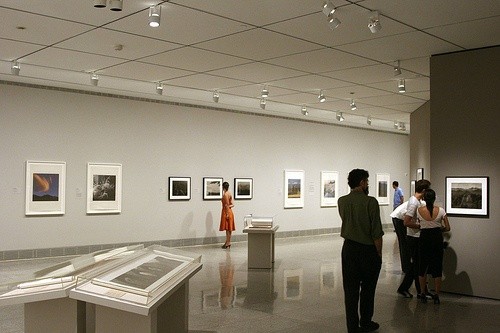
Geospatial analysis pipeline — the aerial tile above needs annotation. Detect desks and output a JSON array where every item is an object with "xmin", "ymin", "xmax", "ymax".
[{"xmin": 243, "ymin": 225, "xmax": 279, "ymax": 268}]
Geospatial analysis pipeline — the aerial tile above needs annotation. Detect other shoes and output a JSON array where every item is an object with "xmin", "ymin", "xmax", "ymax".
[
  {"xmin": 360, "ymin": 321, "xmax": 379, "ymax": 332},
  {"xmin": 348, "ymin": 326, "xmax": 360, "ymax": 333}
]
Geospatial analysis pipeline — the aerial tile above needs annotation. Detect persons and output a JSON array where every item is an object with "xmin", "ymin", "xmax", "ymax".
[
  {"xmin": 393, "ymin": 179, "xmax": 450, "ymax": 304},
  {"xmin": 338, "ymin": 168, "xmax": 385, "ymax": 333},
  {"xmin": 219, "ymin": 182, "xmax": 235, "ymax": 249}
]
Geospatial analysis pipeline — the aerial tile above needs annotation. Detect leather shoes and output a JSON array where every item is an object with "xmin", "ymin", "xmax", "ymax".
[
  {"xmin": 397, "ymin": 289, "xmax": 413, "ymax": 298},
  {"xmin": 418, "ymin": 291, "xmax": 433, "ymax": 298}
]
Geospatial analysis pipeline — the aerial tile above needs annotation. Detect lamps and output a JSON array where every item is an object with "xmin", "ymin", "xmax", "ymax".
[
  {"xmin": 149, "ymin": 2, "xmax": 161, "ymax": 27},
  {"xmin": 11, "ymin": 42, "xmax": 406, "ymax": 132},
  {"xmin": 93, "ymin": 0, "xmax": 122, "ymax": 12},
  {"xmin": 367, "ymin": 8, "xmax": 382, "ymax": 35},
  {"xmin": 321, "ymin": 0, "xmax": 341, "ymax": 29}
]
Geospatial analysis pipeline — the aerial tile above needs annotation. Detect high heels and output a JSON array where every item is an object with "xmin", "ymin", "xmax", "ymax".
[
  {"xmin": 421, "ymin": 293, "xmax": 427, "ymax": 303},
  {"xmin": 433, "ymin": 296, "xmax": 440, "ymax": 305},
  {"xmin": 222, "ymin": 243, "xmax": 231, "ymax": 248}
]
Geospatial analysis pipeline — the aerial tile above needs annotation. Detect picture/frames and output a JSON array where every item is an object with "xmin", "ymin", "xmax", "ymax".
[
  {"xmin": 168, "ymin": 177, "xmax": 192, "ymax": 200},
  {"xmin": 410, "ymin": 168, "xmax": 423, "ymax": 196},
  {"xmin": 234, "ymin": 178, "xmax": 253, "ymax": 200},
  {"xmin": 284, "ymin": 170, "xmax": 305, "ymax": 208},
  {"xmin": 376, "ymin": 173, "xmax": 390, "ymax": 205},
  {"xmin": 25, "ymin": 160, "xmax": 66, "ymax": 215},
  {"xmin": 321, "ymin": 171, "xmax": 340, "ymax": 207},
  {"xmin": 203, "ymin": 177, "xmax": 223, "ymax": 200},
  {"xmin": 86, "ymin": 163, "xmax": 121, "ymax": 215},
  {"xmin": 445, "ymin": 176, "xmax": 490, "ymax": 218}
]
